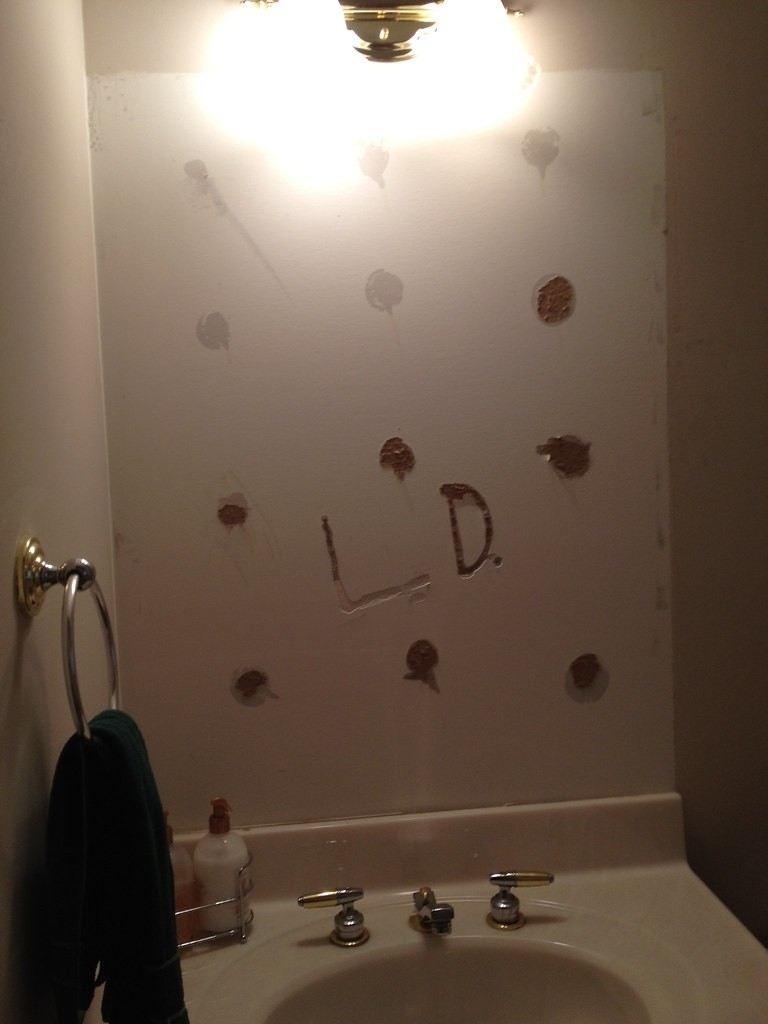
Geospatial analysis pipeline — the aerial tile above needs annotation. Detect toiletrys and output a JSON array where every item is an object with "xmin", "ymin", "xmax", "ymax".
[{"xmin": 153, "ymin": 799, "xmax": 250, "ymax": 947}]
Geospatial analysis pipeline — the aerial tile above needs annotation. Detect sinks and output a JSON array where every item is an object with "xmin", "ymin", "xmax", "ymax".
[{"xmin": 84, "ymin": 793, "xmax": 767, "ymax": 1024}]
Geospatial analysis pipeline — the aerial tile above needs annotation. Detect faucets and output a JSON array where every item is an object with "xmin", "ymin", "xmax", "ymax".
[{"xmin": 411, "ymin": 887, "xmax": 454, "ymax": 938}]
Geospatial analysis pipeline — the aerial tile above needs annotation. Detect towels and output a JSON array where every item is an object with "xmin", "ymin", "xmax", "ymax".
[{"xmin": 42, "ymin": 709, "xmax": 187, "ymax": 1022}]
